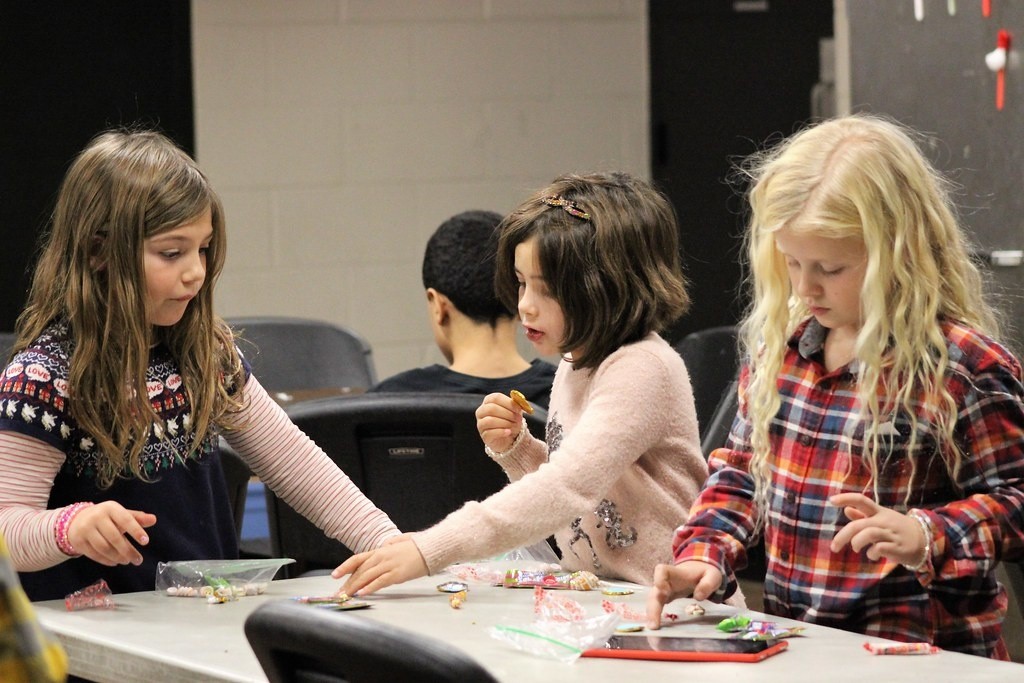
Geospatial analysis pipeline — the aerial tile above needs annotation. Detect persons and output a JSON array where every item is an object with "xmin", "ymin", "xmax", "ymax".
[
  {"xmin": 0, "ymin": 133, "xmax": 406, "ymax": 600},
  {"xmin": 647, "ymin": 115, "xmax": 1024, "ymax": 663},
  {"xmin": 331, "ymin": 171, "xmax": 748, "ymax": 609},
  {"xmin": 366, "ymin": 210, "xmax": 559, "ymax": 410}
]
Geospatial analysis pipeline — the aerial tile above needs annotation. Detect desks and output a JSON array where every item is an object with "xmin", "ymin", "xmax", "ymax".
[{"xmin": 27, "ymin": 559, "xmax": 1024, "ymax": 683}]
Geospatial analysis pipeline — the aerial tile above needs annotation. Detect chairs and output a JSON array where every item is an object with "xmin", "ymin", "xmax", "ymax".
[
  {"xmin": 678, "ymin": 323, "xmax": 769, "ymax": 586},
  {"xmin": 243, "ymin": 597, "xmax": 498, "ymax": 683},
  {"xmin": 263, "ymin": 390, "xmax": 547, "ymax": 575},
  {"xmin": 224, "ymin": 315, "xmax": 378, "ymax": 390}
]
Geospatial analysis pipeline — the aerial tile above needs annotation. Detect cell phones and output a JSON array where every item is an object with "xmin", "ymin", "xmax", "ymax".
[{"xmin": 580, "ymin": 634, "xmax": 789, "ymax": 664}]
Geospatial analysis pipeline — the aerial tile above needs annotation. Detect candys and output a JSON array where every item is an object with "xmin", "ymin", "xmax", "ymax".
[
  {"xmin": 448, "ymin": 567, "xmax": 596, "ymax": 609},
  {"xmin": 863, "ymin": 640, "xmax": 940, "ymax": 656},
  {"xmin": 716, "ymin": 615, "xmax": 804, "ymax": 641},
  {"xmin": 164, "ymin": 576, "xmax": 265, "ymax": 605}
]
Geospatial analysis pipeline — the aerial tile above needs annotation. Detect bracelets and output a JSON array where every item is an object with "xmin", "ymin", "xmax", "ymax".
[
  {"xmin": 903, "ymin": 514, "xmax": 929, "ymax": 571},
  {"xmin": 56, "ymin": 502, "xmax": 96, "ymax": 556}
]
